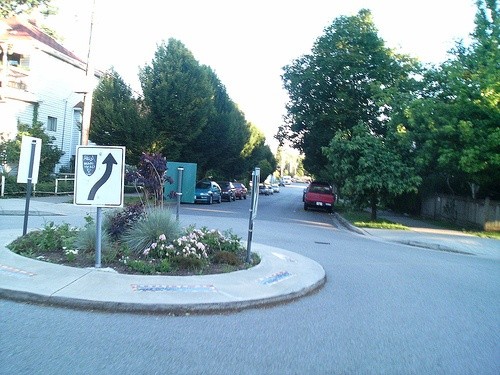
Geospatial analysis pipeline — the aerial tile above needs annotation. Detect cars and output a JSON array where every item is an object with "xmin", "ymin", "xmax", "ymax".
[
  {"xmin": 194, "ymin": 180, "xmax": 221, "ymax": 204},
  {"xmin": 304, "ymin": 182, "xmax": 335, "ymax": 213},
  {"xmin": 216, "ymin": 181, "xmax": 236, "ymax": 202},
  {"xmin": 259, "ymin": 176, "xmax": 291, "ymax": 196},
  {"xmin": 232, "ymin": 183, "xmax": 247, "ymax": 200}
]
